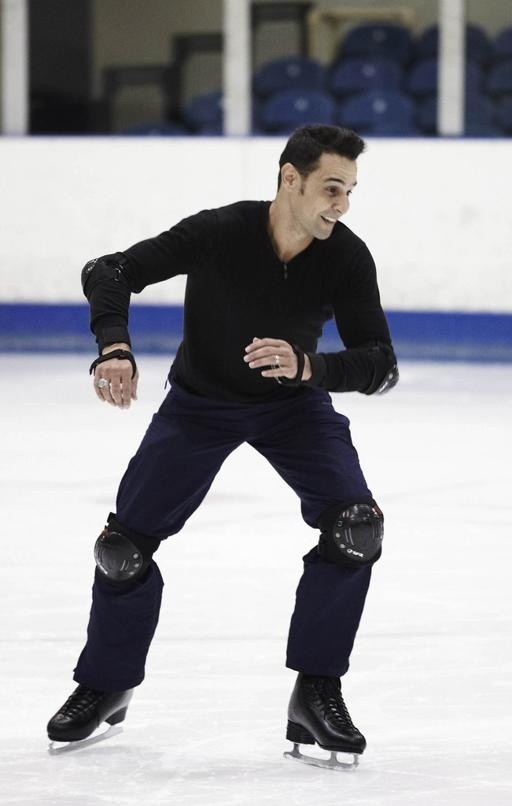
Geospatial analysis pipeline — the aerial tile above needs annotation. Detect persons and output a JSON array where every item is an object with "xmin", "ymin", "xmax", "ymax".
[{"xmin": 48, "ymin": 124, "xmax": 397, "ymax": 752}]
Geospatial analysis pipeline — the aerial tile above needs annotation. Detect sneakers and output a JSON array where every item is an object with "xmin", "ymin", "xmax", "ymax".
[
  {"xmin": 47, "ymin": 684, "xmax": 135, "ymax": 741},
  {"xmin": 286, "ymin": 672, "xmax": 365, "ymax": 754}
]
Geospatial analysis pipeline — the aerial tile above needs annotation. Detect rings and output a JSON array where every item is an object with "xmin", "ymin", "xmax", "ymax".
[
  {"xmin": 275, "ymin": 355, "xmax": 280, "ymax": 366},
  {"xmin": 97, "ymin": 378, "xmax": 110, "ymax": 388}
]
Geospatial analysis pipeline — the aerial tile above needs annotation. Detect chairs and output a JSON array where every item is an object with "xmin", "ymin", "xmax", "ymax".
[{"xmin": 148, "ymin": 21, "xmax": 512, "ymax": 139}]
